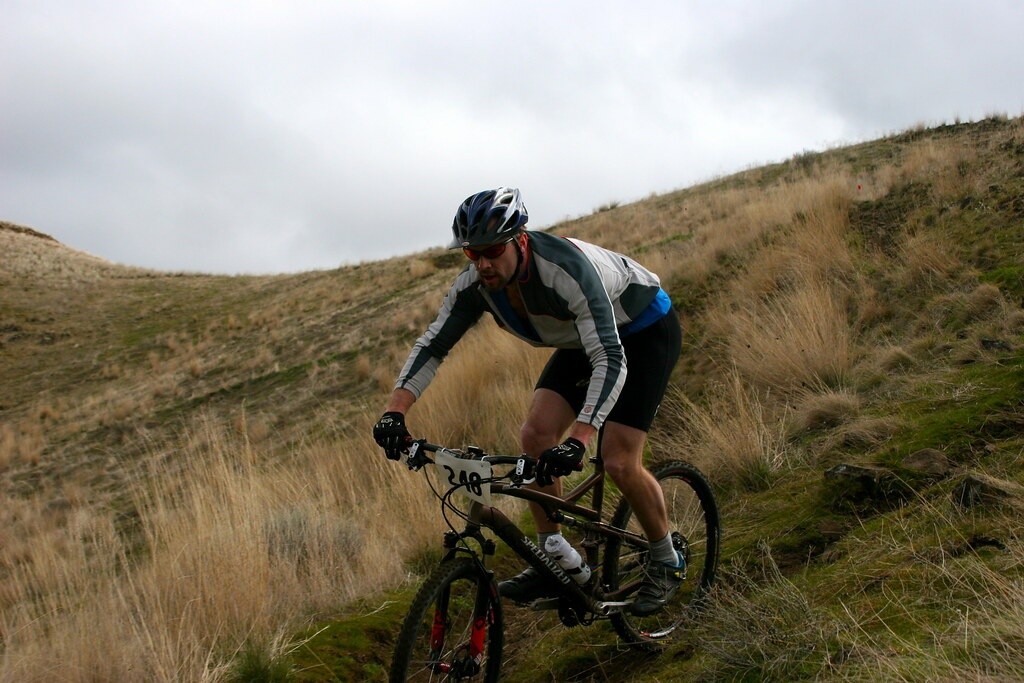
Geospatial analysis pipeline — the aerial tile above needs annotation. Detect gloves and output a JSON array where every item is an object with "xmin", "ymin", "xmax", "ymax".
[
  {"xmin": 535, "ymin": 437, "xmax": 586, "ymax": 487},
  {"xmin": 373, "ymin": 412, "xmax": 412, "ymax": 461}
]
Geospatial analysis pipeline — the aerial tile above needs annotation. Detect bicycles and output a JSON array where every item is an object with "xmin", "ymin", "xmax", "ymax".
[{"xmin": 372, "ymin": 422, "xmax": 721, "ymax": 683}]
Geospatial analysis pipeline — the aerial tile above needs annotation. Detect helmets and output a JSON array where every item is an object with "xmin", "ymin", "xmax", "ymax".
[{"xmin": 448, "ymin": 187, "xmax": 528, "ymax": 250}]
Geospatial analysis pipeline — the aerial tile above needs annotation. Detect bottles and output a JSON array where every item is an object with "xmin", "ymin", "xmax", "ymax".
[{"xmin": 544, "ymin": 534, "xmax": 592, "ymax": 585}]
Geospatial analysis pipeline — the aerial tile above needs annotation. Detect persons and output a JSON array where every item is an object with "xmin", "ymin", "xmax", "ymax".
[{"xmin": 373, "ymin": 189, "xmax": 688, "ymax": 617}]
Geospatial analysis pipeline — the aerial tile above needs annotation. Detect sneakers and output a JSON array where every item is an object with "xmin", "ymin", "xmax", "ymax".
[
  {"xmin": 497, "ymin": 561, "xmax": 566, "ymax": 603},
  {"xmin": 631, "ymin": 551, "xmax": 688, "ymax": 617}
]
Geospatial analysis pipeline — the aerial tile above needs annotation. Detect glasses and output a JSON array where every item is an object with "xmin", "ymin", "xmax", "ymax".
[{"xmin": 463, "ymin": 237, "xmax": 513, "ymax": 261}]
